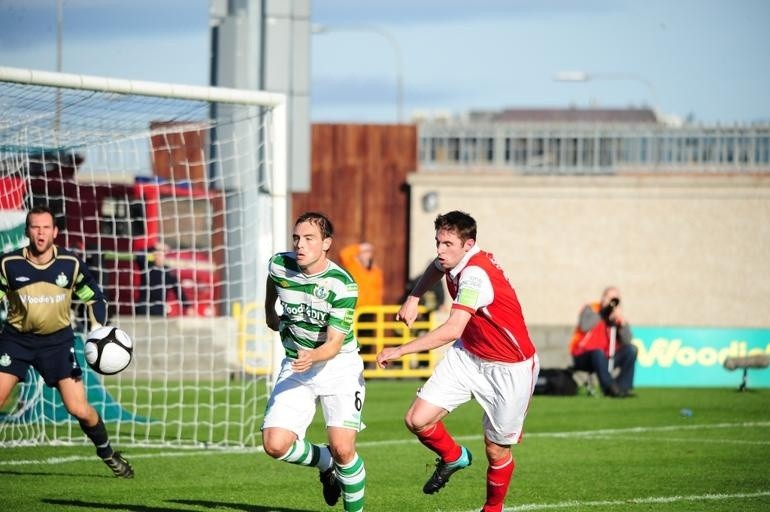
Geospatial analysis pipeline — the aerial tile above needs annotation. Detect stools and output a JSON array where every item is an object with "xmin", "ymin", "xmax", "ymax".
[{"xmin": 564, "ymin": 366, "xmax": 599, "ymax": 397}]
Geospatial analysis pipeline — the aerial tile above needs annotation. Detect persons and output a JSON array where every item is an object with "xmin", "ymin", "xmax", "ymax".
[
  {"xmin": 260, "ymin": 212, "xmax": 367, "ymax": 511},
  {"xmin": 135, "ymin": 242, "xmax": 197, "ymax": 317},
  {"xmin": 571, "ymin": 286, "xmax": 638, "ymax": 396},
  {"xmin": 0, "ymin": 204, "xmax": 135, "ymax": 480},
  {"xmin": 339, "ymin": 240, "xmax": 384, "ymax": 380},
  {"xmin": 376, "ymin": 211, "xmax": 542, "ymax": 512}
]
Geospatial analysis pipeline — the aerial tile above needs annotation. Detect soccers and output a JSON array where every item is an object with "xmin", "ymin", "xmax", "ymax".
[{"xmin": 85, "ymin": 327, "xmax": 132, "ymax": 374}]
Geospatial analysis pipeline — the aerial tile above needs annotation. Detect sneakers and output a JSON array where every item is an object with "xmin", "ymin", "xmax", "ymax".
[
  {"xmin": 421, "ymin": 441, "xmax": 474, "ymax": 495},
  {"xmin": 102, "ymin": 448, "xmax": 137, "ymax": 482},
  {"xmin": 318, "ymin": 442, "xmax": 341, "ymax": 508}
]
